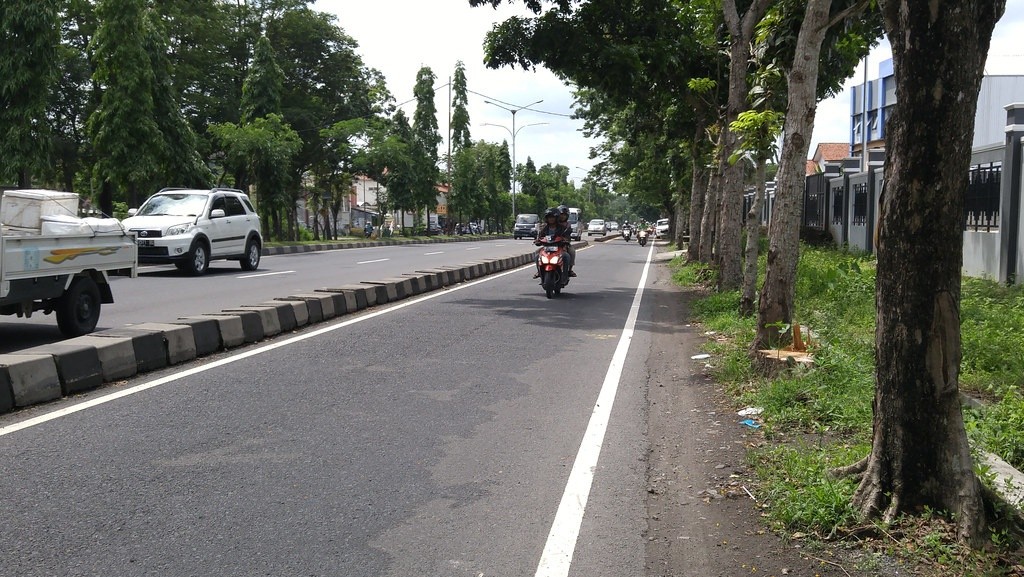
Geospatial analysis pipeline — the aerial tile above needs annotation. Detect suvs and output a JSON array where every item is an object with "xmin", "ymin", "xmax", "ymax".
[
  {"xmin": 656, "ymin": 218, "xmax": 669, "ymax": 239},
  {"xmin": 513, "ymin": 213, "xmax": 542, "ymax": 240},
  {"xmin": 117, "ymin": 187, "xmax": 263, "ymax": 276}
]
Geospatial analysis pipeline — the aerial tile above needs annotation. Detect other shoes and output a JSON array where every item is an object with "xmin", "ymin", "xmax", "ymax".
[
  {"xmin": 568, "ymin": 271, "xmax": 577, "ymax": 277},
  {"xmin": 533, "ymin": 272, "xmax": 540, "ymax": 279},
  {"xmin": 568, "ymin": 278, "xmax": 570, "ymax": 281},
  {"xmin": 539, "ymin": 281, "xmax": 542, "ymax": 285}
]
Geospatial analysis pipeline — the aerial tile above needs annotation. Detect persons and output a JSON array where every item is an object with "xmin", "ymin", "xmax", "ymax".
[
  {"xmin": 632, "ymin": 219, "xmax": 657, "ymax": 244},
  {"xmin": 622, "ymin": 220, "xmax": 633, "ymax": 237},
  {"xmin": 533, "ymin": 204, "xmax": 578, "ymax": 278},
  {"xmin": 363, "ymin": 222, "xmax": 373, "ymax": 237}
]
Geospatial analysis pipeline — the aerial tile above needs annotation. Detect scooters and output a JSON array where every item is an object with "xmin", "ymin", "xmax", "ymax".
[
  {"xmin": 533, "ymin": 234, "xmax": 573, "ymax": 299},
  {"xmin": 364, "ymin": 224, "xmax": 373, "ymax": 238},
  {"xmin": 621, "ymin": 226, "xmax": 633, "ymax": 242},
  {"xmin": 632, "ymin": 226, "xmax": 653, "ymax": 247}
]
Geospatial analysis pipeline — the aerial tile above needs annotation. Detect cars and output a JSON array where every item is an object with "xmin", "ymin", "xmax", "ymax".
[
  {"xmin": 611, "ymin": 222, "xmax": 619, "ymax": 231},
  {"xmin": 606, "ymin": 222, "xmax": 612, "ymax": 232},
  {"xmin": 586, "ymin": 219, "xmax": 607, "ymax": 236},
  {"xmin": 454, "ymin": 223, "xmax": 482, "ymax": 234}
]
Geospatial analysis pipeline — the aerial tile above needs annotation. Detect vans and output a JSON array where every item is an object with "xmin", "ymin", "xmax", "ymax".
[{"xmin": 569, "ymin": 207, "xmax": 584, "ymax": 241}]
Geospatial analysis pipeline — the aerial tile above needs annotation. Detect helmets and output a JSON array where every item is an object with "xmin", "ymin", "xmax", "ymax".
[
  {"xmin": 633, "ymin": 219, "xmax": 656, "ymax": 226},
  {"xmin": 544, "ymin": 207, "xmax": 561, "ymax": 216},
  {"xmin": 366, "ymin": 221, "xmax": 370, "ymax": 224},
  {"xmin": 557, "ymin": 205, "xmax": 571, "ymax": 215},
  {"xmin": 625, "ymin": 220, "xmax": 629, "ymax": 223}
]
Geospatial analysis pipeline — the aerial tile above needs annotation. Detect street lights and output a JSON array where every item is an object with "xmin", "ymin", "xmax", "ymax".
[{"xmin": 480, "ymin": 96, "xmax": 550, "ymax": 219}]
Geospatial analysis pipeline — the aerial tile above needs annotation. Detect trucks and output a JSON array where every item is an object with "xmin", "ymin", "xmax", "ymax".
[{"xmin": 0, "ymin": 232, "xmax": 139, "ymax": 342}]
